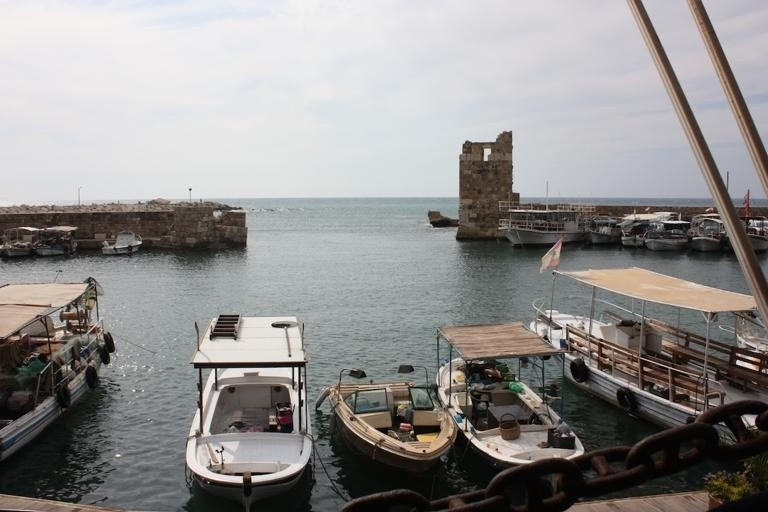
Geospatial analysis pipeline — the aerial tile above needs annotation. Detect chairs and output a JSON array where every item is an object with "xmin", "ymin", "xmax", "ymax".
[
  {"xmin": 470, "ymin": 390, "xmax": 492, "ymax": 425},
  {"xmin": 352, "ymin": 397, "xmax": 370, "ymax": 412}
]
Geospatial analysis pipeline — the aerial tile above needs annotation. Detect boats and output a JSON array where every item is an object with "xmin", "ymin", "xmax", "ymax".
[
  {"xmin": 435, "ymin": 319, "xmax": 585, "ymax": 470},
  {"xmin": 718, "ymin": 321, "xmax": 768, "ymax": 376},
  {"xmin": 100, "ymin": 229, "xmax": 143, "ymax": 256},
  {"xmin": 496, "ymin": 201, "xmax": 693, "ymax": 252},
  {"xmin": 0, "ymin": 275, "xmax": 116, "ymax": 465},
  {"xmin": 315, "ymin": 362, "xmax": 460, "ymax": 474},
  {"xmin": 0, "ymin": 224, "xmax": 79, "ymax": 259},
  {"xmin": 724, "ymin": 212, "xmax": 768, "ymax": 253},
  {"xmin": 184, "ymin": 311, "xmax": 315, "ymax": 512},
  {"xmin": 688, "ymin": 212, "xmax": 726, "ymax": 252},
  {"xmin": 525, "ymin": 262, "xmax": 768, "ymax": 446}
]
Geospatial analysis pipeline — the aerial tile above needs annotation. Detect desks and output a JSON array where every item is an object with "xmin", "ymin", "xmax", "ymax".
[{"xmin": 487, "ymin": 405, "xmax": 531, "ymax": 429}]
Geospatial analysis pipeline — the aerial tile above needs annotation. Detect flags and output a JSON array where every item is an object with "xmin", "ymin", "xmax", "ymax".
[{"xmin": 539, "ymin": 237, "xmax": 563, "ymax": 272}]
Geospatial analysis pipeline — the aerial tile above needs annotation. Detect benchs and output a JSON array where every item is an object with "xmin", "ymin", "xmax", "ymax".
[
  {"xmin": 566, "ymin": 319, "xmax": 768, "ymax": 413},
  {"xmin": 392, "ymin": 389, "xmax": 412, "ymax": 408}
]
